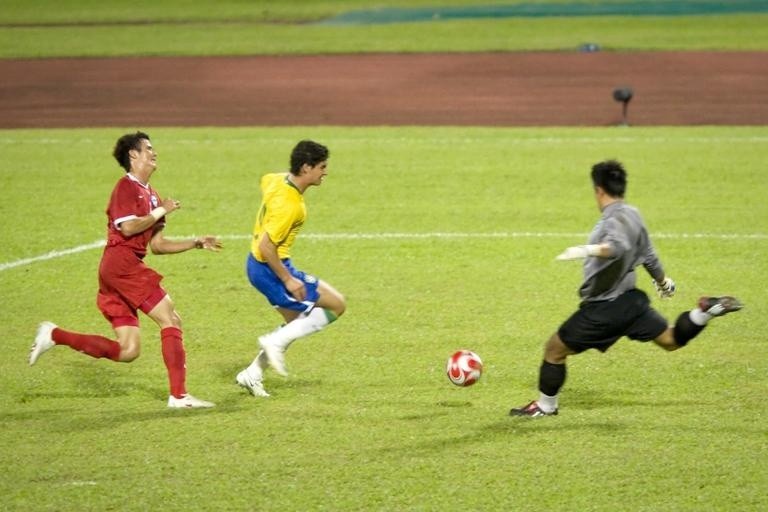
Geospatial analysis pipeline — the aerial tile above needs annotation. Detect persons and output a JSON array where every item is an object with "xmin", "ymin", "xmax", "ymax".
[
  {"xmin": 235, "ymin": 139, "xmax": 346, "ymax": 398},
  {"xmin": 27, "ymin": 129, "xmax": 225, "ymax": 411},
  {"xmin": 508, "ymin": 159, "xmax": 742, "ymax": 419}
]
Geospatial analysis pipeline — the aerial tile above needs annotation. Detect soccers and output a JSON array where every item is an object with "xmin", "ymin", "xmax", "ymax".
[{"xmin": 448, "ymin": 349, "xmax": 483, "ymax": 387}]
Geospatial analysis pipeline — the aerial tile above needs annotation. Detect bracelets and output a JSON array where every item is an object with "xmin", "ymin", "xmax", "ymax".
[
  {"xmin": 149, "ymin": 206, "xmax": 167, "ymax": 221},
  {"xmin": 193, "ymin": 238, "xmax": 204, "ymax": 249}
]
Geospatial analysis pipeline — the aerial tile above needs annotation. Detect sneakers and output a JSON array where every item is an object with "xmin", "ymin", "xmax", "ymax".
[
  {"xmin": 236, "ymin": 369, "xmax": 270, "ymax": 396},
  {"xmin": 258, "ymin": 335, "xmax": 287, "ymax": 376},
  {"xmin": 167, "ymin": 393, "xmax": 216, "ymax": 408},
  {"xmin": 29, "ymin": 321, "xmax": 58, "ymax": 366},
  {"xmin": 511, "ymin": 400, "xmax": 558, "ymax": 416},
  {"xmin": 699, "ymin": 296, "xmax": 743, "ymax": 316}
]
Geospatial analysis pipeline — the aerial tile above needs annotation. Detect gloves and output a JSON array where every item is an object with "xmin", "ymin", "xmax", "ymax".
[
  {"xmin": 555, "ymin": 244, "xmax": 600, "ymax": 260},
  {"xmin": 652, "ymin": 279, "xmax": 677, "ymax": 299}
]
